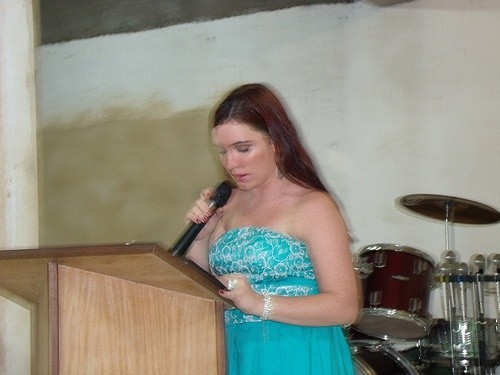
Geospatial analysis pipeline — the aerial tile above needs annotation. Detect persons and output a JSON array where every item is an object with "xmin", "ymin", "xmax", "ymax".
[{"xmin": 183, "ymin": 82, "xmax": 359, "ymax": 375}]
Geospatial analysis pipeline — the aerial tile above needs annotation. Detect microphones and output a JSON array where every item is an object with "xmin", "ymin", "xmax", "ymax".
[{"xmin": 167, "ymin": 180, "xmax": 232, "ymax": 257}]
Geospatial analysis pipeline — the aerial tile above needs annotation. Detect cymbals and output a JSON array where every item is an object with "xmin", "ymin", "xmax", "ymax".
[{"xmin": 399, "ymin": 192, "xmax": 500, "ymax": 229}]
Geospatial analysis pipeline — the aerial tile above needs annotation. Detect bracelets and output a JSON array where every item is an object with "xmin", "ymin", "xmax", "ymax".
[{"xmin": 258, "ymin": 292, "xmax": 272, "ymax": 321}]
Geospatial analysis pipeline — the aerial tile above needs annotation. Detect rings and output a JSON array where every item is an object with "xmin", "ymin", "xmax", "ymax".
[{"xmin": 227, "ymin": 279, "xmax": 237, "ymax": 291}]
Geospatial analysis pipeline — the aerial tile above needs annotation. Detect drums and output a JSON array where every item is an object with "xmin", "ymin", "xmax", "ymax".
[
  {"xmin": 347, "ymin": 343, "xmax": 420, "ymax": 375},
  {"xmin": 350, "ymin": 242, "xmax": 434, "ymax": 340},
  {"xmin": 438, "ymin": 319, "xmax": 499, "ymax": 361}
]
